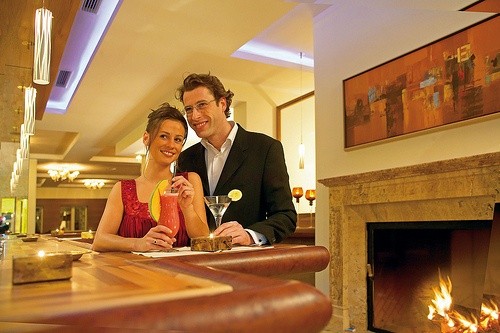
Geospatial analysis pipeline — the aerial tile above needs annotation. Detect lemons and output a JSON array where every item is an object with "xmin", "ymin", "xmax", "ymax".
[{"xmin": 228, "ymin": 189, "xmax": 242, "ymax": 201}]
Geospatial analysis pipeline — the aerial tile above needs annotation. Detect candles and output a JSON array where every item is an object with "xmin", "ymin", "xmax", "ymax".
[
  {"xmin": 81, "ymin": 229, "xmax": 95, "ymax": 239},
  {"xmin": 191, "ymin": 235, "xmax": 232, "ymax": 252},
  {"xmin": 11, "ymin": 251, "xmax": 72, "ymax": 285},
  {"xmin": 51, "ymin": 229, "xmax": 64, "ymax": 236}
]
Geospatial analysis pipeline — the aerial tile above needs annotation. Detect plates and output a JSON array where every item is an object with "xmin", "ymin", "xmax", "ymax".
[
  {"xmin": 26, "ymin": 251, "xmax": 91, "ymax": 261},
  {"xmin": 21, "ymin": 238, "xmax": 38, "ymax": 242},
  {"xmin": 17, "ymin": 235, "xmax": 26, "ymax": 237}
]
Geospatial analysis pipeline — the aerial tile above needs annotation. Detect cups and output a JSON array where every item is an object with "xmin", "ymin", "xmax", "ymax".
[
  {"xmin": 306, "ymin": 190, "xmax": 316, "ymax": 200},
  {"xmin": 292, "ymin": 187, "xmax": 303, "ymax": 198}
]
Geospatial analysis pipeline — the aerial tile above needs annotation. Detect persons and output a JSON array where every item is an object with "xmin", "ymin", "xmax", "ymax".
[
  {"xmin": 92, "ymin": 103, "xmax": 210, "ymax": 251},
  {"xmin": 176, "ymin": 73, "xmax": 297, "ymax": 247},
  {"xmin": 0, "ymin": 216, "xmax": 4, "ymax": 225}
]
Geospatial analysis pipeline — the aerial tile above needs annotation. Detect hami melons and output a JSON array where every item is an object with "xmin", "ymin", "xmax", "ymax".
[{"xmin": 149, "ymin": 179, "xmax": 168, "ymax": 222}]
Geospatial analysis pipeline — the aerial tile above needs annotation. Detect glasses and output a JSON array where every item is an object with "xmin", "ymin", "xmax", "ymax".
[{"xmin": 182, "ymin": 99, "xmax": 216, "ymax": 114}]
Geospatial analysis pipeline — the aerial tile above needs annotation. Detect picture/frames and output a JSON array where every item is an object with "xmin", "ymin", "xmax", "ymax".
[{"xmin": 343, "ymin": 13, "xmax": 500, "ymax": 152}]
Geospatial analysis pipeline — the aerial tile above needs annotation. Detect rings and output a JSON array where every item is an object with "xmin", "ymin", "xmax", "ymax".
[{"xmin": 154, "ymin": 240, "xmax": 157, "ymax": 244}]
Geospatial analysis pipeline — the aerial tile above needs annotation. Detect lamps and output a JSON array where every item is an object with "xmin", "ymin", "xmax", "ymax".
[
  {"xmin": 50, "ymin": 169, "xmax": 79, "ymax": 182},
  {"xmin": 293, "ymin": 188, "xmax": 315, "ymax": 229},
  {"xmin": 11, "ymin": 85, "xmax": 36, "ymax": 192},
  {"xmin": 85, "ymin": 179, "xmax": 104, "ymax": 190},
  {"xmin": 299, "ymin": 52, "xmax": 303, "ymax": 170},
  {"xmin": 33, "ymin": 0, "xmax": 52, "ymax": 85}
]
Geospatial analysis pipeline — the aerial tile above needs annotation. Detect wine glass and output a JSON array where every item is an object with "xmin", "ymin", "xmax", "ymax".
[
  {"xmin": 158, "ymin": 187, "xmax": 180, "ymax": 251},
  {"xmin": 203, "ymin": 195, "xmax": 232, "ymax": 228}
]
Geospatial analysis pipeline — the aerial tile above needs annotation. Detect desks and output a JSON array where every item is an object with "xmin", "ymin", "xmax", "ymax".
[{"xmin": 0, "ymin": 231, "xmax": 330, "ymax": 333}]
